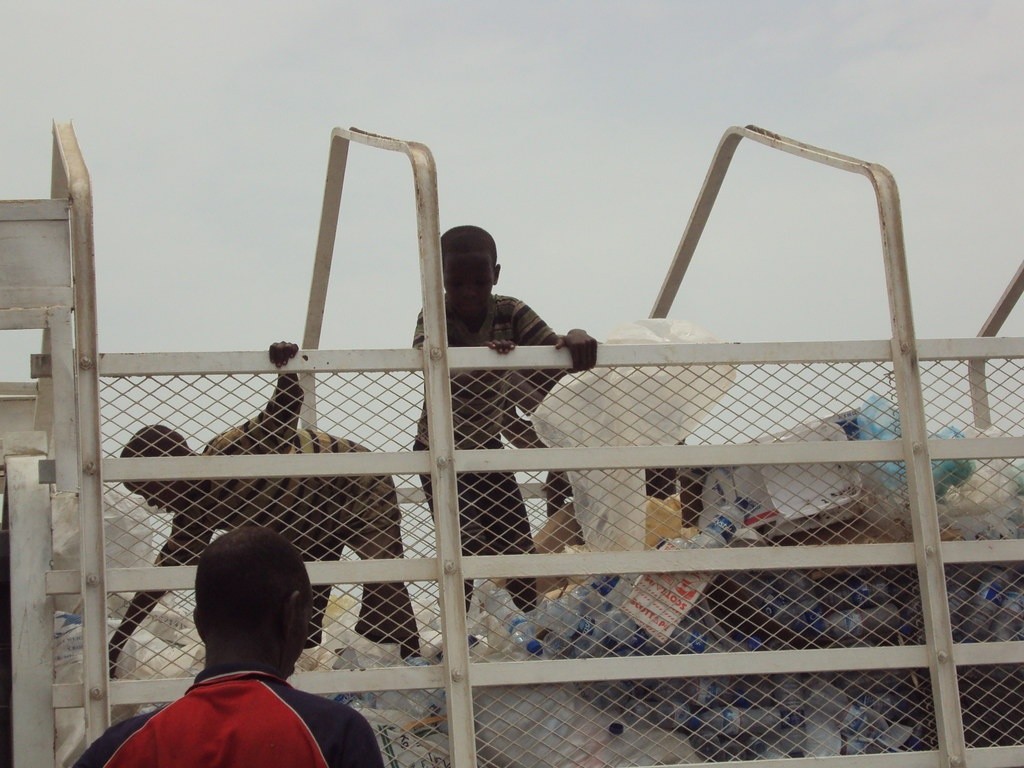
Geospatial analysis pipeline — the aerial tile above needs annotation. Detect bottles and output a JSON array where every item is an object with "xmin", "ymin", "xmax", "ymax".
[{"xmin": 330, "ymin": 495, "xmax": 1024, "ymax": 756}]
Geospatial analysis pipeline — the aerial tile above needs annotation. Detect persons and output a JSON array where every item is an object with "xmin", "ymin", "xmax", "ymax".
[
  {"xmin": 73, "ymin": 528, "xmax": 385, "ymax": 768},
  {"xmin": 412, "ymin": 225, "xmax": 711, "ymax": 645},
  {"xmin": 108, "ymin": 341, "xmax": 421, "ymax": 679}
]
